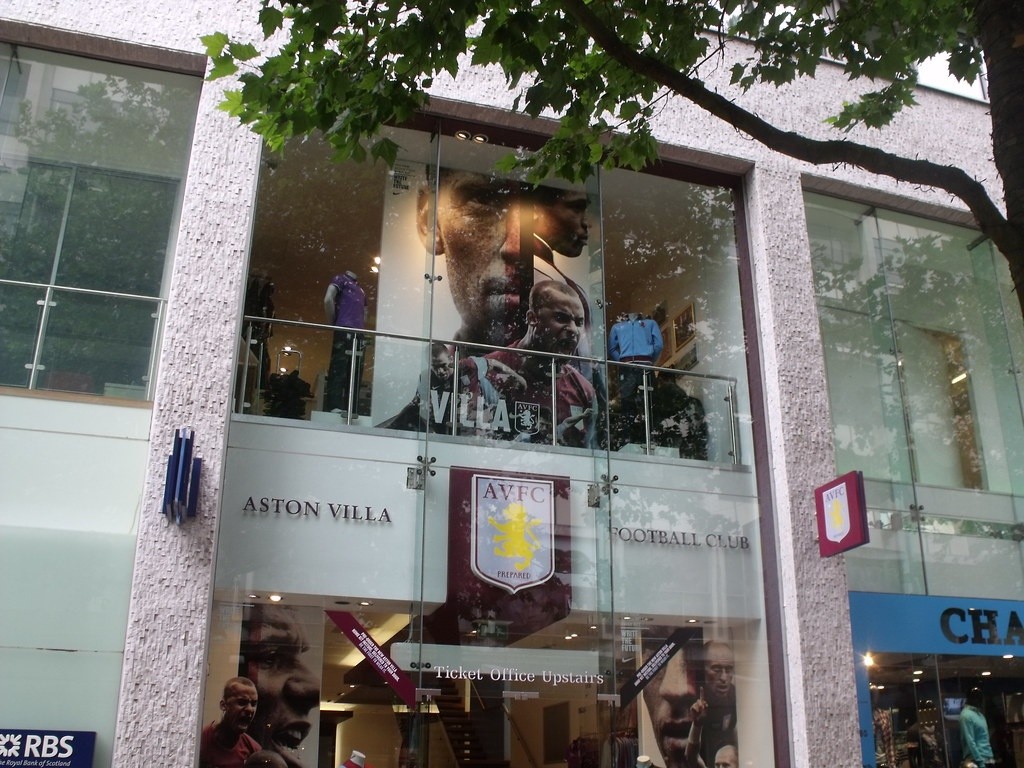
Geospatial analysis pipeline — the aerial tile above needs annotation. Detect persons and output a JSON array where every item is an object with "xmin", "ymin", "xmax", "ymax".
[
  {"xmin": 324, "ymin": 271, "xmax": 370, "ymax": 414},
  {"xmin": 200, "ymin": 600, "xmax": 319, "ymax": 768},
  {"xmin": 608, "ymin": 312, "xmax": 663, "ymax": 442},
  {"xmin": 959, "ymin": 691, "xmax": 995, "ymax": 768},
  {"xmin": 389, "ymin": 165, "xmax": 597, "ymax": 443},
  {"xmin": 641, "ymin": 626, "xmax": 740, "ymax": 768}
]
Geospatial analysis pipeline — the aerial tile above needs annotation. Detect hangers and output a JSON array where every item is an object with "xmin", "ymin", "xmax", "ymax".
[{"xmin": 574, "ymin": 726, "xmax": 636, "ymax": 743}]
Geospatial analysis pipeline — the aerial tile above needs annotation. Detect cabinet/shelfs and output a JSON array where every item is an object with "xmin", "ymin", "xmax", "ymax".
[{"xmin": 231, "ymin": 321, "xmax": 263, "ymax": 415}]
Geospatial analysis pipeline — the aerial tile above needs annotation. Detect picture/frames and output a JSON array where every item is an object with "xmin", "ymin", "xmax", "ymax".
[
  {"xmin": 673, "ymin": 301, "xmax": 696, "ymax": 352},
  {"xmin": 668, "ymin": 341, "xmax": 700, "ymax": 381},
  {"xmin": 654, "ymin": 322, "xmax": 673, "ymax": 367},
  {"xmin": 652, "ymin": 300, "xmax": 668, "ymax": 329}
]
